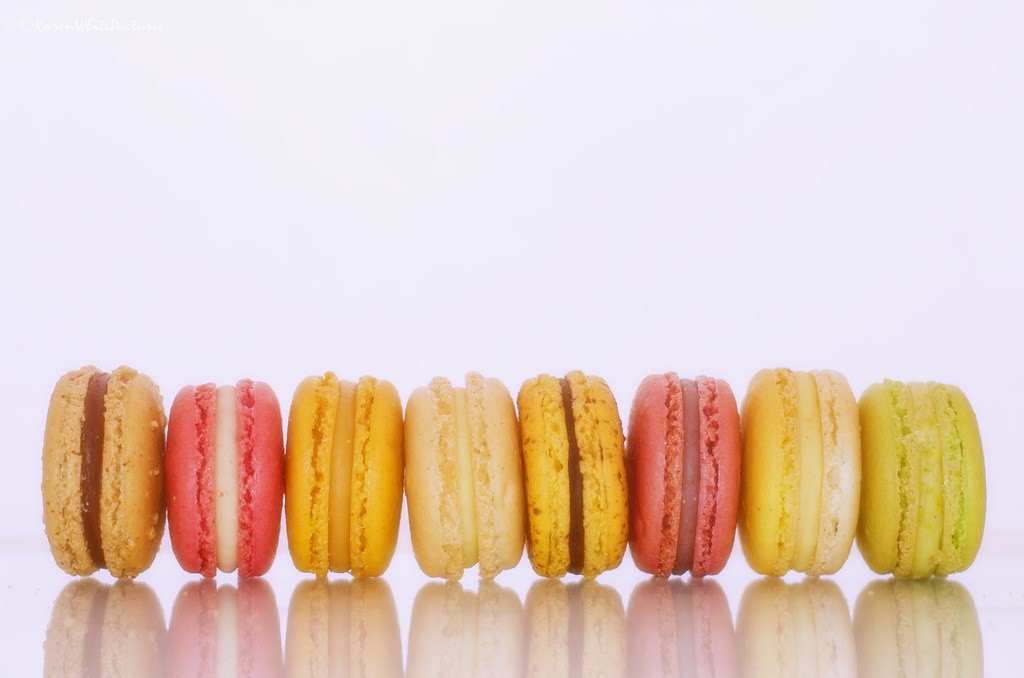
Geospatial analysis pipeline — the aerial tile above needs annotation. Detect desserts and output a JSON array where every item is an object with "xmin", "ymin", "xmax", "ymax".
[
  {"xmin": 39, "ymin": 364, "xmax": 987, "ymax": 576},
  {"xmin": 41, "ymin": 581, "xmax": 983, "ymax": 678}
]
